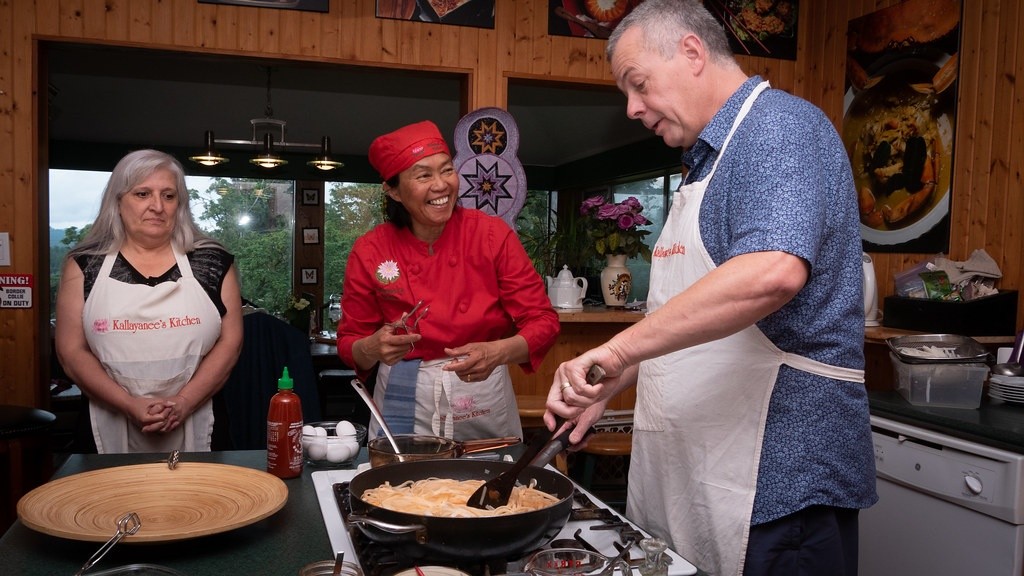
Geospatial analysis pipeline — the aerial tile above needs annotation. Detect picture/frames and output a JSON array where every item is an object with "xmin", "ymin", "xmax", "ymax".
[
  {"xmin": 302, "ymin": 227, "xmax": 321, "ymax": 245},
  {"xmin": 300, "ymin": 268, "xmax": 318, "ymax": 285},
  {"xmin": 302, "ymin": 188, "xmax": 320, "ymax": 206}
]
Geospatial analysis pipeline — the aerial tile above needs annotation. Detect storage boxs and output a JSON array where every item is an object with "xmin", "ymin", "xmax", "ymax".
[
  {"xmin": 890, "ymin": 351, "xmax": 991, "ymax": 410},
  {"xmin": 883, "ymin": 289, "xmax": 1021, "ymax": 336}
]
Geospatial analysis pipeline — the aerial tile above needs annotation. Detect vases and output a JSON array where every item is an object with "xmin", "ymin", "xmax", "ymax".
[
  {"xmin": 600, "ymin": 254, "xmax": 631, "ymax": 306},
  {"xmin": 290, "ymin": 319, "xmax": 309, "ymax": 338}
]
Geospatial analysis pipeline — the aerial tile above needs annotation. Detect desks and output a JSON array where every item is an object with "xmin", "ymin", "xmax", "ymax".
[{"xmin": 311, "ymin": 342, "xmax": 342, "ymax": 421}]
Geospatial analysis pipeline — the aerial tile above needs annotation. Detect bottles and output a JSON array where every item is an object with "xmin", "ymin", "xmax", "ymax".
[
  {"xmin": 267, "ymin": 367, "xmax": 303, "ymax": 479},
  {"xmin": 329, "ymin": 294, "xmax": 342, "ymax": 339}
]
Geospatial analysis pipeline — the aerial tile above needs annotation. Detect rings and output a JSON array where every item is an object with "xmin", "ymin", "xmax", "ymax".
[
  {"xmin": 468, "ymin": 374, "xmax": 470, "ymax": 382},
  {"xmin": 560, "ymin": 382, "xmax": 571, "ymax": 392}
]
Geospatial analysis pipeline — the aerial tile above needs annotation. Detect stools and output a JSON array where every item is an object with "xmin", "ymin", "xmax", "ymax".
[
  {"xmin": 584, "ymin": 433, "xmax": 632, "ymax": 507},
  {"xmin": 516, "ymin": 394, "xmax": 568, "ymax": 477}
]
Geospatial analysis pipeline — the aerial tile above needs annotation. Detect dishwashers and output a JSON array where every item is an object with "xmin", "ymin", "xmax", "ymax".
[{"xmin": 858, "ymin": 415, "xmax": 1024, "ymax": 576}]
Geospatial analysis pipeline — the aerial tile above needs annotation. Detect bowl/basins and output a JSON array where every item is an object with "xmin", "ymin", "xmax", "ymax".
[
  {"xmin": 303, "ymin": 421, "xmax": 367, "ymax": 469},
  {"xmin": 842, "ymin": 86, "xmax": 953, "ymax": 245}
]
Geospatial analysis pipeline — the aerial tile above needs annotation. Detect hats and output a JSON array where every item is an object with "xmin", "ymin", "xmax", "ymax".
[{"xmin": 369, "ymin": 120, "xmax": 451, "ymax": 181}]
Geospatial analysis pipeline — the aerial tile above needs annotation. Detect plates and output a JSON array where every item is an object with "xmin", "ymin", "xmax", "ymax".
[{"xmin": 988, "ymin": 376, "xmax": 1024, "ymax": 403}]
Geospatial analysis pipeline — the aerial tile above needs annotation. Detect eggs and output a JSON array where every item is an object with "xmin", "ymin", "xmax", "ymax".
[{"xmin": 299, "ymin": 421, "xmax": 360, "ymax": 463}]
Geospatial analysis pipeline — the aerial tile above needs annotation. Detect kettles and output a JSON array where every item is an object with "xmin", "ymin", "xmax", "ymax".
[{"xmin": 861, "ymin": 250, "xmax": 880, "ymax": 326}]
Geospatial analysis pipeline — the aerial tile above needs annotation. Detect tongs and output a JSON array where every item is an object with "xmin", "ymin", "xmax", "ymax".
[
  {"xmin": 385, "ymin": 299, "xmax": 430, "ymax": 353},
  {"xmin": 75, "ymin": 512, "xmax": 142, "ymax": 576}
]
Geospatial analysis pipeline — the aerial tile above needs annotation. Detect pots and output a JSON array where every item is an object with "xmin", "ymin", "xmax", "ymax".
[
  {"xmin": 349, "ymin": 459, "xmax": 574, "ymax": 564},
  {"xmin": 369, "ymin": 434, "xmax": 521, "ymax": 468}
]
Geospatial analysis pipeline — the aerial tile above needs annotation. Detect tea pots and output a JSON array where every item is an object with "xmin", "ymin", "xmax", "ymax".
[{"xmin": 546, "ymin": 264, "xmax": 588, "ymax": 313}]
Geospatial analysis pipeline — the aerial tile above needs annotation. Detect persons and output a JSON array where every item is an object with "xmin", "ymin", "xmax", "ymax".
[
  {"xmin": 543, "ymin": 0, "xmax": 879, "ymax": 576},
  {"xmin": 55, "ymin": 150, "xmax": 244, "ymax": 452},
  {"xmin": 336, "ymin": 120, "xmax": 559, "ymax": 445}
]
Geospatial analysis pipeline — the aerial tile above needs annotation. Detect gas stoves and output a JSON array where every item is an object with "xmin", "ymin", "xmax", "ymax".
[{"xmin": 311, "ymin": 468, "xmax": 698, "ymax": 576}]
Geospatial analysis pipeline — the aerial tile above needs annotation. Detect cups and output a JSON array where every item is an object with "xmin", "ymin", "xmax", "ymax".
[{"xmin": 300, "ymin": 560, "xmax": 362, "ymax": 576}]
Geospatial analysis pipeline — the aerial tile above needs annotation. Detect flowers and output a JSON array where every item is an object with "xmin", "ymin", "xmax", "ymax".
[
  {"xmin": 283, "ymin": 296, "xmax": 316, "ymax": 327},
  {"xmin": 579, "ymin": 195, "xmax": 652, "ymax": 263}
]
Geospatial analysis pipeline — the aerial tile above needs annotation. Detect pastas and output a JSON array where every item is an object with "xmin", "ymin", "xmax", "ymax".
[{"xmin": 360, "ymin": 476, "xmax": 561, "ymax": 518}]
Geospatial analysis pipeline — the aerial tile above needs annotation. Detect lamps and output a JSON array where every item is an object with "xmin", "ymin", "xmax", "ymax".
[{"xmin": 188, "ymin": 63, "xmax": 344, "ymax": 170}]
